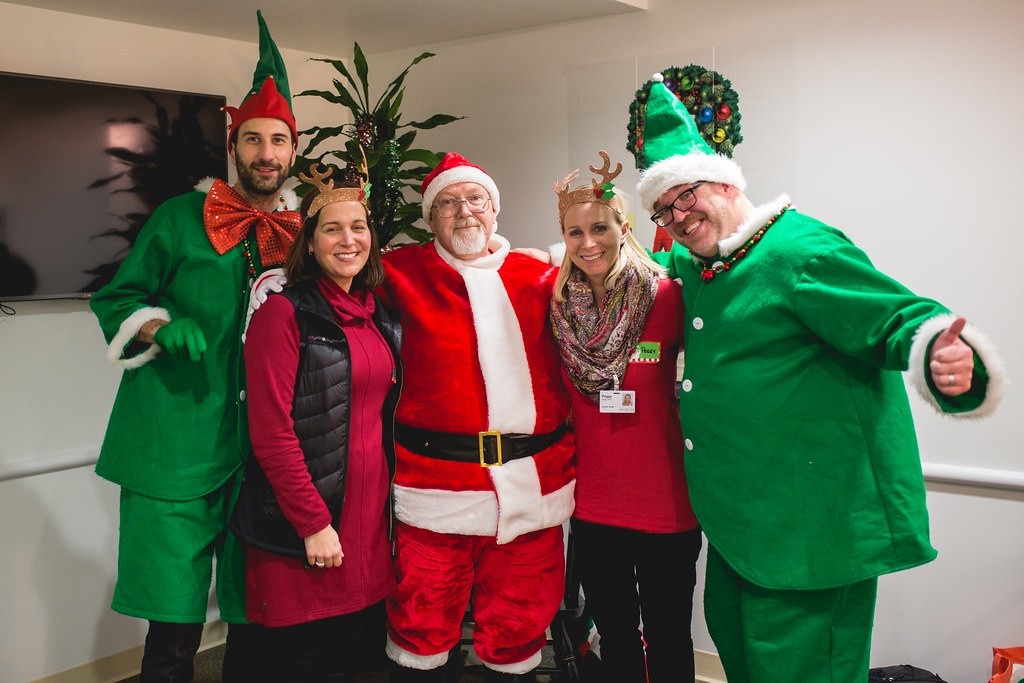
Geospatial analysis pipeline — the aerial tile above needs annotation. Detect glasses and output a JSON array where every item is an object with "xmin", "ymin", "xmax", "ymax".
[
  {"xmin": 431, "ymin": 194, "xmax": 491, "ymax": 218},
  {"xmin": 650, "ymin": 180, "xmax": 706, "ymax": 228}
]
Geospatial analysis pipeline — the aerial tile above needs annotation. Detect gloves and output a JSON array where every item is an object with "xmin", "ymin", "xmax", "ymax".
[
  {"xmin": 153, "ymin": 315, "xmax": 206, "ymax": 363},
  {"xmin": 250, "ymin": 275, "xmax": 288, "ymax": 311}
]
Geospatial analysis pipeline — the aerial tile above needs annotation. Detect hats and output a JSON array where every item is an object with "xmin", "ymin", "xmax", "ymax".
[
  {"xmin": 420, "ymin": 151, "xmax": 500, "ymax": 226},
  {"xmin": 219, "ymin": 8, "xmax": 299, "ymax": 152},
  {"xmin": 634, "ymin": 72, "xmax": 747, "ymax": 217}
]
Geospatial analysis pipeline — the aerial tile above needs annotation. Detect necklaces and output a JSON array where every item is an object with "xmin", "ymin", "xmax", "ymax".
[
  {"xmin": 697, "ymin": 203, "xmax": 791, "ymax": 283},
  {"xmin": 244, "ymin": 194, "xmax": 289, "ymax": 280}
]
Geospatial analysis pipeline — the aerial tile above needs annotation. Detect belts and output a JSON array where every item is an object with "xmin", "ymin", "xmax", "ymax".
[{"xmin": 393, "ymin": 419, "xmax": 570, "ymax": 468}]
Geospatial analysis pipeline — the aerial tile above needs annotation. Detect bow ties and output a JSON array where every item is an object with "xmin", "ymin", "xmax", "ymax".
[{"xmin": 203, "ymin": 178, "xmax": 304, "ymax": 266}]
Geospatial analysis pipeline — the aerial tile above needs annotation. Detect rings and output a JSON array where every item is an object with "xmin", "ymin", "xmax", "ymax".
[
  {"xmin": 316, "ymin": 562, "xmax": 325, "ymax": 566},
  {"xmin": 949, "ymin": 374, "xmax": 954, "ymax": 386}
]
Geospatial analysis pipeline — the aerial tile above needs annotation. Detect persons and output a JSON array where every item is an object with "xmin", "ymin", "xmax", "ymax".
[
  {"xmin": 547, "ymin": 147, "xmax": 703, "ymax": 683},
  {"xmin": 509, "ymin": 71, "xmax": 1006, "ymax": 683},
  {"xmin": 229, "ymin": 144, "xmax": 402, "ymax": 682},
  {"xmin": 89, "ymin": 79, "xmax": 305, "ymax": 683},
  {"xmin": 250, "ymin": 150, "xmax": 576, "ymax": 681}
]
